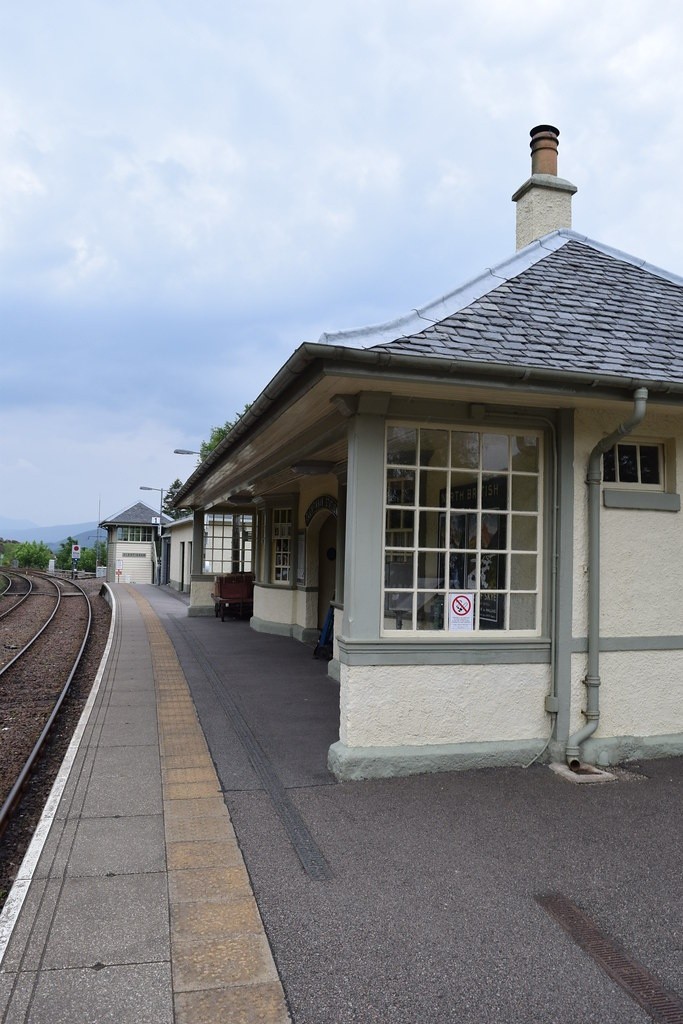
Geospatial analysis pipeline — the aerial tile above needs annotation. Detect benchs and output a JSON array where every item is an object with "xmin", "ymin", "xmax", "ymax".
[{"xmin": 212, "ymin": 574, "xmax": 255, "ymax": 622}]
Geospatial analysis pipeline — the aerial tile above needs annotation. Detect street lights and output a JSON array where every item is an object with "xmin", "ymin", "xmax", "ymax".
[{"xmin": 140, "ymin": 486, "xmax": 169, "ymax": 565}]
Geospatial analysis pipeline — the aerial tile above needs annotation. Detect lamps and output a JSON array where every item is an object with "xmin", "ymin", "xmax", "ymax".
[
  {"xmin": 290, "ymin": 460, "xmax": 334, "ymax": 476},
  {"xmin": 229, "ymin": 495, "xmax": 253, "ymax": 503}
]
